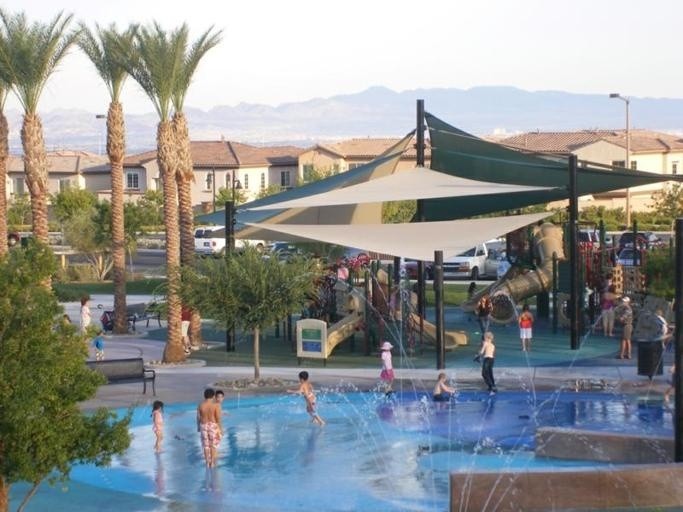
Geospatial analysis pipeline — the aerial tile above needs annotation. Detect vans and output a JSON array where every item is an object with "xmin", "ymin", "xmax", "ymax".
[
  {"xmin": 272, "ymin": 242, "xmax": 370, "ymax": 266},
  {"xmin": 194, "ymin": 226, "xmax": 266, "ymax": 259}
]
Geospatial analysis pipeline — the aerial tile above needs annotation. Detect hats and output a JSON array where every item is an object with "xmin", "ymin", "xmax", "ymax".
[
  {"xmin": 622, "ymin": 297, "xmax": 635, "ymax": 304},
  {"xmin": 379, "ymin": 340, "xmax": 396, "ymax": 351}
]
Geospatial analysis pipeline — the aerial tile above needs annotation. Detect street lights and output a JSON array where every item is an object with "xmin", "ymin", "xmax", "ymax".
[
  {"xmin": 609, "ymin": 93, "xmax": 631, "ymax": 227},
  {"xmin": 206, "ymin": 167, "xmax": 215, "ymax": 212},
  {"xmin": 233, "ymin": 170, "xmax": 242, "ymax": 206}
]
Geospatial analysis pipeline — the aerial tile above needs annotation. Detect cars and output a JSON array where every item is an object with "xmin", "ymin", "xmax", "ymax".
[{"xmin": 406, "ymin": 229, "xmax": 676, "ymax": 280}]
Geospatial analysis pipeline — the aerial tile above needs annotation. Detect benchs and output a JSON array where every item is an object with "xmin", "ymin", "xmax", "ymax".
[
  {"xmin": 84, "ymin": 359, "xmax": 156, "ymax": 396},
  {"xmin": 103, "ymin": 309, "xmax": 135, "ymax": 331},
  {"xmin": 145, "ymin": 309, "xmax": 162, "ymax": 329}
]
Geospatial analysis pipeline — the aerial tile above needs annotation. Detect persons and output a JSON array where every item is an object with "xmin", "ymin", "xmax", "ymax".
[
  {"xmin": 213, "ymin": 389, "xmax": 223, "ymax": 407},
  {"xmin": 154, "ymin": 455, "xmax": 164, "ymax": 498},
  {"xmin": 655, "ymin": 305, "xmax": 668, "ymax": 347},
  {"xmin": 388, "ymin": 285, "xmax": 398, "ymax": 321},
  {"xmin": 90, "ymin": 329, "xmax": 106, "ymax": 359},
  {"xmin": 79, "ymin": 296, "xmax": 91, "ymax": 338},
  {"xmin": 473, "ymin": 293, "xmax": 493, "ymax": 339},
  {"xmin": 196, "ymin": 387, "xmax": 222, "ymax": 466},
  {"xmin": 198, "ymin": 466, "xmax": 219, "ymax": 509},
  {"xmin": 295, "ymin": 428, "xmax": 321, "ymax": 468},
  {"xmin": 662, "ymin": 333, "xmax": 676, "ymax": 405},
  {"xmin": 286, "ymin": 371, "xmax": 326, "ymax": 428},
  {"xmin": 376, "ymin": 341, "xmax": 394, "ymax": 396},
  {"xmin": 181, "ymin": 304, "xmax": 192, "ymax": 354},
  {"xmin": 518, "ymin": 303, "xmax": 534, "ymax": 353},
  {"xmin": 617, "ymin": 295, "xmax": 632, "ymax": 359},
  {"xmin": 336, "ymin": 262, "xmax": 350, "ymax": 284},
  {"xmin": 150, "ymin": 398, "xmax": 165, "ymax": 454},
  {"xmin": 433, "ymin": 372, "xmax": 456, "ymax": 402},
  {"xmin": 601, "ymin": 284, "xmax": 621, "ymax": 339},
  {"xmin": 473, "ymin": 331, "xmax": 500, "ymax": 393}
]
